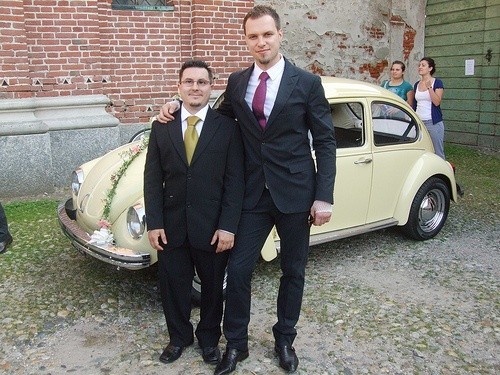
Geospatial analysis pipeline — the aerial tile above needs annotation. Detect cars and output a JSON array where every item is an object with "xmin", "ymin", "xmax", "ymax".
[{"xmin": 59, "ymin": 77, "xmax": 465, "ymax": 270}]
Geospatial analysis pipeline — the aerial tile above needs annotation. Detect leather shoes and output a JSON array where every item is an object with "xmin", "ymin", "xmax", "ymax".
[
  {"xmin": 214, "ymin": 346, "xmax": 250, "ymax": 375},
  {"xmin": 275, "ymin": 342, "xmax": 299, "ymax": 372},
  {"xmin": 159, "ymin": 343, "xmax": 193, "ymax": 363},
  {"xmin": 0, "ymin": 235, "xmax": 13, "ymax": 253},
  {"xmin": 202, "ymin": 341, "xmax": 221, "ymax": 365}
]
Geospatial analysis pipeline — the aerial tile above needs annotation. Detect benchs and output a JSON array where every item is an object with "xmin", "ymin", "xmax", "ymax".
[{"xmin": 333, "ymin": 123, "xmax": 403, "ymax": 145}]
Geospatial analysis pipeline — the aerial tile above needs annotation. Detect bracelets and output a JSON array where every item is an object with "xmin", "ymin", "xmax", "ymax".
[{"xmin": 428, "ymin": 87, "xmax": 432, "ymax": 90}]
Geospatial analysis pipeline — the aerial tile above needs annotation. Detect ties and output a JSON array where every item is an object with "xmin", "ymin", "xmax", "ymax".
[
  {"xmin": 252, "ymin": 72, "xmax": 270, "ymax": 132},
  {"xmin": 182, "ymin": 116, "xmax": 201, "ymax": 166}
]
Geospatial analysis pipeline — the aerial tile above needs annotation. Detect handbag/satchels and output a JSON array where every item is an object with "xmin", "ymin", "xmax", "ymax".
[{"xmin": 372, "ymin": 105, "xmax": 382, "ymax": 117}]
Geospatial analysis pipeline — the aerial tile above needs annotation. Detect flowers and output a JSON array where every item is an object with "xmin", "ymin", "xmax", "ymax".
[{"xmin": 84, "ymin": 218, "xmax": 115, "ymax": 250}]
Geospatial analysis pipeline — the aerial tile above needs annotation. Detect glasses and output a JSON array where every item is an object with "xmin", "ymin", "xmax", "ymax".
[
  {"xmin": 180, "ymin": 79, "xmax": 212, "ymax": 87},
  {"xmin": 307, "ymin": 215, "xmax": 316, "ymax": 227}
]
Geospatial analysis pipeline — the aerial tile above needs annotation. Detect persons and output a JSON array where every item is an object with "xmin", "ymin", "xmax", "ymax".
[
  {"xmin": 157, "ymin": 5, "xmax": 336, "ymax": 375},
  {"xmin": 144, "ymin": 60, "xmax": 245, "ymax": 364},
  {"xmin": 0, "ymin": 202, "xmax": 13, "ymax": 254},
  {"xmin": 413, "ymin": 57, "xmax": 444, "ymax": 160},
  {"xmin": 376, "ymin": 61, "xmax": 414, "ymax": 119}
]
